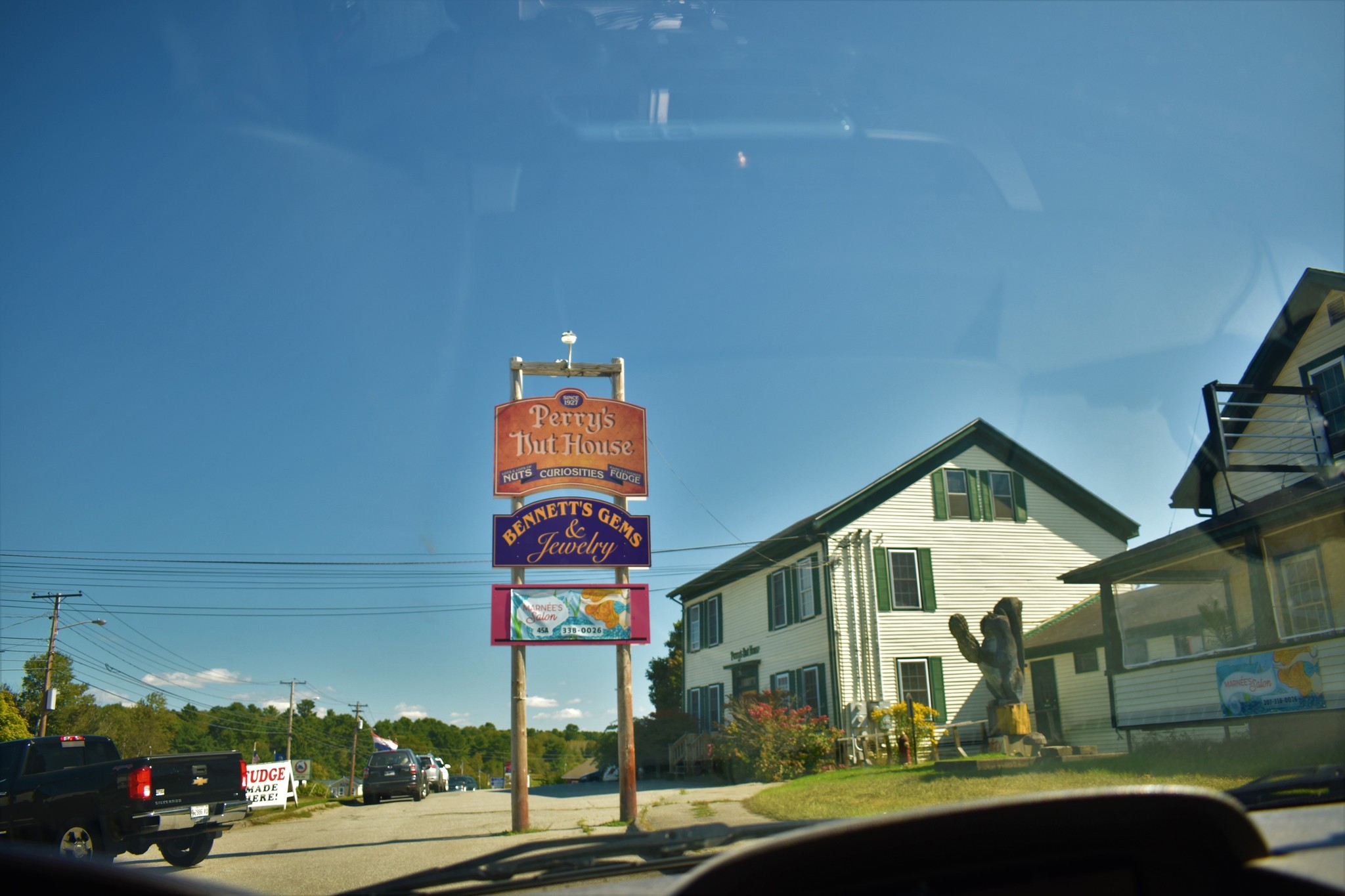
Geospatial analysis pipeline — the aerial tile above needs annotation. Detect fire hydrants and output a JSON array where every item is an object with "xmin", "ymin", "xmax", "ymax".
[{"xmin": 897, "ymin": 732, "xmax": 912, "ymax": 765}]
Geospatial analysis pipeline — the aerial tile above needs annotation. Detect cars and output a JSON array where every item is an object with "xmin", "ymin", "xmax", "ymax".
[
  {"xmin": 578, "ymin": 768, "xmax": 601, "ymax": 784},
  {"xmin": 448, "ymin": 775, "xmax": 478, "ymax": 791}
]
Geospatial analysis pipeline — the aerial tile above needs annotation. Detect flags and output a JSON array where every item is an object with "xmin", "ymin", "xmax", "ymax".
[
  {"xmin": 371, "ymin": 731, "xmax": 398, "ymax": 750},
  {"xmin": 274, "ymin": 752, "xmax": 286, "ymax": 760},
  {"xmin": 253, "ymin": 749, "xmax": 260, "ymax": 763}
]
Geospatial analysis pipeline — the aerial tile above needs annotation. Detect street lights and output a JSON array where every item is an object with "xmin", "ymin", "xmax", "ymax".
[
  {"xmin": 38, "ymin": 618, "xmax": 106, "ymax": 737},
  {"xmin": 286, "ymin": 696, "xmax": 319, "ymax": 759},
  {"xmin": 349, "ymin": 728, "xmax": 376, "ymax": 796}
]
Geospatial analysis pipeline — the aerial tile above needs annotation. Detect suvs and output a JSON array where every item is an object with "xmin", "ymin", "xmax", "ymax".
[{"xmin": 362, "ymin": 748, "xmax": 451, "ymax": 805}]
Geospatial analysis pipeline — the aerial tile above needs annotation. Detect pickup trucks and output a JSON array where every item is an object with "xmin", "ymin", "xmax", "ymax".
[{"xmin": 0, "ymin": 734, "xmax": 255, "ymax": 867}]
